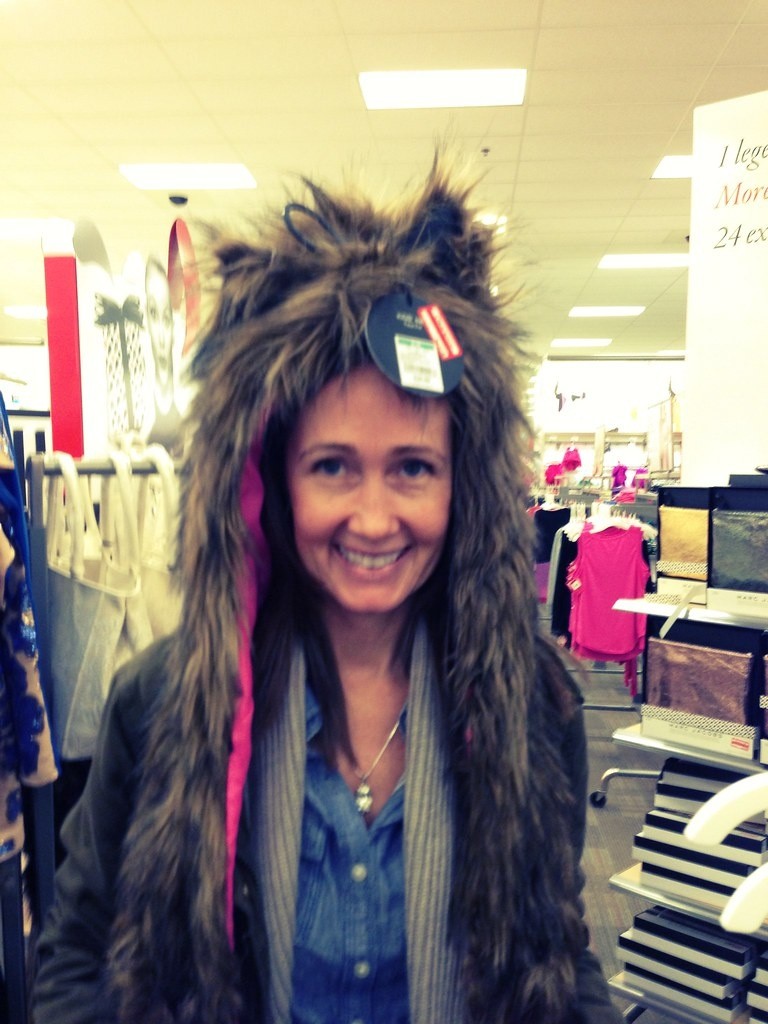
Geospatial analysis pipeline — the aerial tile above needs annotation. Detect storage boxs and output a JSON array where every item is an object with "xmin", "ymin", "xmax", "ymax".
[
  {"xmin": 644, "ymin": 634, "xmax": 756, "ymax": 727},
  {"xmin": 615, "ymin": 756, "xmax": 768, "ymax": 1024}
]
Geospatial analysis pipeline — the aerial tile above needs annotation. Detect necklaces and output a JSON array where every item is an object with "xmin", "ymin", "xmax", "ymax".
[{"xmin": 344, "ymin": 723, "xmax": 399, "ymax": 813}]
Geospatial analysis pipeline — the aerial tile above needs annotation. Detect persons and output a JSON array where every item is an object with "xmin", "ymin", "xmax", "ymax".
[
  {"xmin": 144, "ymin": 256, "xmax": 186, "ymax": 460},
  {"xmin": 29, "ymin": 172, "xmax": 626, "ymax": 1024}
]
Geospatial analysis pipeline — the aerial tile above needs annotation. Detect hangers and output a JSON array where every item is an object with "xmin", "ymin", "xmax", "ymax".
[
  {"xmin": 540, "ymin": 493, "xmax": 587, "ymax": 542},
  {"xmin": 590, "ymin": 501, "xmax": 658, "ymax": 541}
]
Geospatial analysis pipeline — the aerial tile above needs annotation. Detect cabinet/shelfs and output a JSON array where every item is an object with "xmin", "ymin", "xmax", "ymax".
[{"xmin": 605, "ymin": 598, "xmax": 768, "ymax": 1024}]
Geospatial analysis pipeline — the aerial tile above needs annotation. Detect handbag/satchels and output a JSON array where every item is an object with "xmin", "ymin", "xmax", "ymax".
[{"xmin": 25, "ymin": 442, "xmax": 191, "ymax": 760}]
